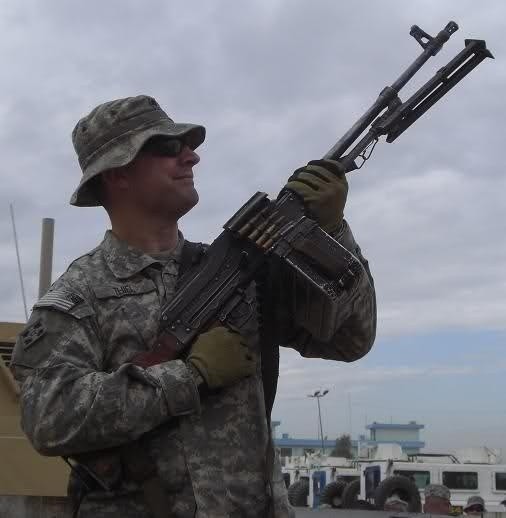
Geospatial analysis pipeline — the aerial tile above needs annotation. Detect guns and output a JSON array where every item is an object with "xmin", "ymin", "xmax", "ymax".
[{"xmin": 63, "ymin": 21, "xmax": 494, "ymax": 493}]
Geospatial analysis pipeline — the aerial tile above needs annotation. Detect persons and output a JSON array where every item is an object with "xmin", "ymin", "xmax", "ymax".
[{"xmin": 9, "ymin": 94, "xmax": 376, "ymax": 517}]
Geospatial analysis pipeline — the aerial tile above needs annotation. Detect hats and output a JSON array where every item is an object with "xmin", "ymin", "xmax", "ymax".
[{"xmin": 69, "ymin": 95, "xmax": 206, "ymax": 207}]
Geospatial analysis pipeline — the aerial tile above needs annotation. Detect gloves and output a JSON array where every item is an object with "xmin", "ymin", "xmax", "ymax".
[
  {"xmin": 186, "ymin": 326, "xmax": 256, "ymax": 390},
  {"xmin": 284, "ymin": 159, "xmax": 348, "ymax": 235}
]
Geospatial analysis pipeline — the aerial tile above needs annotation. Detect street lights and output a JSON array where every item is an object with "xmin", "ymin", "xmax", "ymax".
[{"xmin": 307, "ymin": 388, "xmax": 330, "ymax": 455}]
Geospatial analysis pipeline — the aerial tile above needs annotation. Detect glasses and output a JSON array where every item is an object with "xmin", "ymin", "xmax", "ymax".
[{"xmin": 142, "ymin": 135, "xmax": 184, "ymax": 158}]
